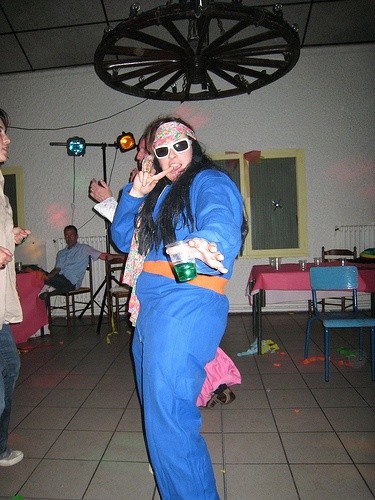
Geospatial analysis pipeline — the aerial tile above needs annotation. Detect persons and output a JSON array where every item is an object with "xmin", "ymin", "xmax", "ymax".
[
  {"xmin": 25, "ymin": 225, "xmax": 107, "ymax": 299},
  {"xmin": 110, "ymin": 114, "xmax": 247, "ymax": 500},
  {"xmin": 87, "ymin": 136, "xmax": 241, "ymax": 405},
  {"xmin": 0, "ymin": 106, "xmax": 32, "ymax": 466}
]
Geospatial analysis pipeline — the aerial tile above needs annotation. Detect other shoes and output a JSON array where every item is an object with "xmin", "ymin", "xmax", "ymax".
[
  {"xmin": 202, "ymin": 387, "xmax": 236, "ymax": 408},
  {"xmin": 38, "ymin": 286, "xmax": 57, "ymax": 299}
]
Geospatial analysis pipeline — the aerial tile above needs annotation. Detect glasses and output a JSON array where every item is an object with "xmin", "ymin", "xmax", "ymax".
[{"xmin": 153, "ymin": 137, "xmax": 193, "ymax": 159}]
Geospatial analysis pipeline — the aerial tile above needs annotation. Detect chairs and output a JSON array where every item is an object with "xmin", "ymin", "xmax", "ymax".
[
  {"xmin": 49, "ymin": 253, "xmax": 131, "ymax": 330},
  {"xmin": 304, "ymin": 247, "xmax": 375, "ymax": 382}
]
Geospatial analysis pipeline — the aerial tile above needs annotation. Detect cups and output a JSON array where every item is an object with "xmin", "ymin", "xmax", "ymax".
[
  {"xmin": 269, "ymin": 256, "xmax": 281, "ymax": 271},
  {"xmin": 313, "ymin": 257, "xmax": 320, "ymax": 267},
  {"xmin": 15, "ymin": 262, "xmax": 22, "ymax": 272},
  {"xmin": 340, "ymin": 257, "xmax": 346, "ymax": 267},
  {"xmin": 299, "ymin": 259, "xmax": 307, "ymax": 272},
  {"xmin": 165, "ymin": 238, "xmax": 197, "ymax": 283}
]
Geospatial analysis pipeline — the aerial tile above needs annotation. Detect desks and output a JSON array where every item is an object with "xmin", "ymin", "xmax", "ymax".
[
  {"xmin": 8, "ymin": 267, "xmax": 50, "ymax": 345},
  {"xmin": 245, "ymin": 262, "xmax": 375, "ymax": 356}
]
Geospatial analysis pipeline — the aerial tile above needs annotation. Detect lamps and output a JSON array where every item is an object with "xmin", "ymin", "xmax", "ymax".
[
  {"xmin": 66, "ymin": 136, "xmax": 86, "ymax": 157},
  {"xmin": 114, "ymin": 131, "xmax": 137, "ymax": 153}
]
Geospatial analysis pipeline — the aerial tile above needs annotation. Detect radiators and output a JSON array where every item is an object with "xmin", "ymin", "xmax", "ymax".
[
  {"xmin": 46, "ymin": 235, "xmax": 127, "ymax": 309},
  {"xmin": 334, "ymin": 223, "xmax": 375, "ymax": 259}
]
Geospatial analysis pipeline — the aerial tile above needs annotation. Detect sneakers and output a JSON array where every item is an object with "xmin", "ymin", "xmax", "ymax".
[{"xmin": 0, "ymin": 449, "xmax": 23, "ymax": 466}]
